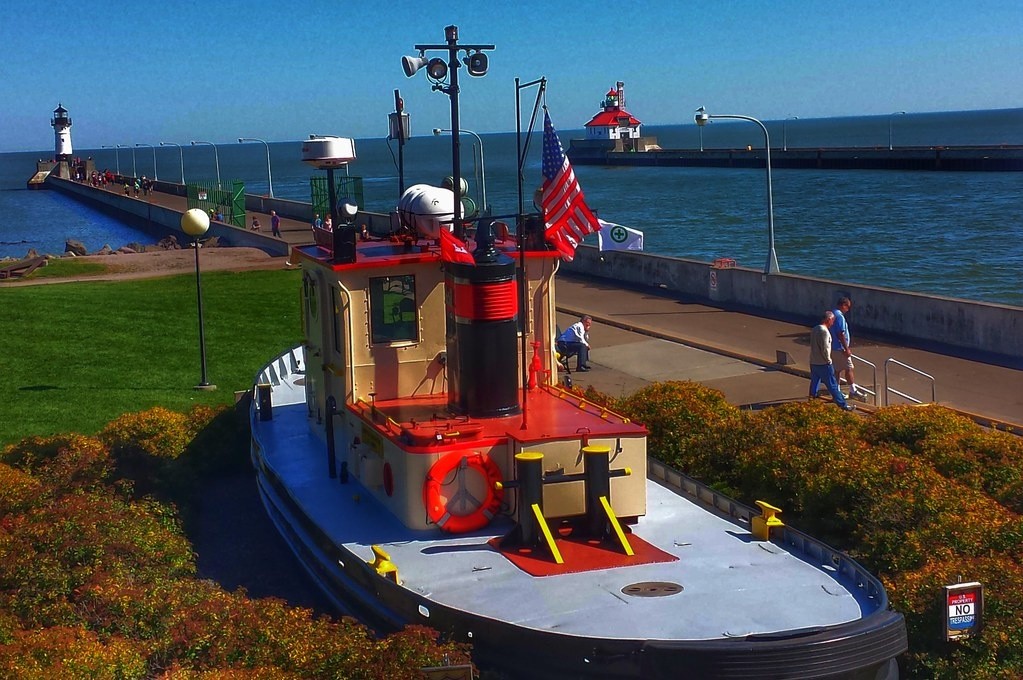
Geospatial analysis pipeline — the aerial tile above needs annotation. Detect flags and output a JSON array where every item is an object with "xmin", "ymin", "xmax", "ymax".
[{"xmin": 542, "ymin": 105, "xmax": 604, "ymax": 264}]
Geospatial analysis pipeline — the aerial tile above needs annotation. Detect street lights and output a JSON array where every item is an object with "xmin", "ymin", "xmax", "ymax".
[
  {"xmin": 693, "ymin": 106, "xmax": 781, "ymax": 276},
  {"xmin": 888, "ymin": 110, "xmax": 906, "ymax": 150},
  {"xmin": 783, "ymin": 116, "xmax": 798, "ymax": 150},
  {"xmin": 238, "ymin": 138, "xmax": 275, "ymax": 198},
  {"xmin": 160, "ymin": 142, "xmax": 186, "ymax": 185},
  {"xmin": 117, "ymin": 145, "xmax": 137, "ymax": 179},
  {"xmin": 432, "ymin": 128, "xmax": 487, "ymax": 212},
  {"xmin": 101, "ymin": 145, "xmax": 119, "ymax": 175},
  {"xmin": 135, "ymin": 143, "xmax": 158, "ymax": 181},
  {"xmin": 181, "ymin": 209, "xmax": 216, "ymax": 391},
  {"xmin": 191, "ymin": 141, "xmax": 221, "ymax": 190}
]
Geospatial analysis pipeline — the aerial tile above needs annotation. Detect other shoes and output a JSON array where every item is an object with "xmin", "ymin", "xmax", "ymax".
[
  {"xmin": 831, "ymin": 393, "xmax": 849, "ymax": 399},
  {"xmin": 844, "ymin": 405, "xmax": 856, "ymax": 410},
  {"xmin": 849, "ymin": 390, "xmax": 868, "ymax": 398},
  {"xmin": 576, "ymin": 366, "xmax": 591, "ymax": 372}
]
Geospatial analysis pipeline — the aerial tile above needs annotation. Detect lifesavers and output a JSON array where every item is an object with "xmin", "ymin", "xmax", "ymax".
[{"xmin": 424, "ymin": 450, "xmax": 505, "ymax": 533}]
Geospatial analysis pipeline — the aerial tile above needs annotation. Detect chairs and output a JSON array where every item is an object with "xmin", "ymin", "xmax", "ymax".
[{"xmin": 556, "ymin": 324, "xmax": 577, "ymax": 374}]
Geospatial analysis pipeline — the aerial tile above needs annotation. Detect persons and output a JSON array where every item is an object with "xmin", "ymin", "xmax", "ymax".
[
  {"xmin": 557, "ymin": 315, "xmax": 593, "ymax": 371},
  {"xmin": 311, "ymin": 213, "xmax": 333, "ymax": 241},
  {"xmin": 359, "ymin": 224, "xmax": 370, "ymax": 241},
  {"xmin": 209, "ymin": 208, "xmax": 223, "ymax": 221},
  {"xmin": 47, "ymin": 157, "xmax": 155, "ymax": 198},
  {"xmin": 251, "ymin": 216, "xmax": 262, "ymax": 233},
  {"xmin": 269, "ymin": 209, "xmax": 283, "ymax": 238},
  {"xmin": 809, "ymin": 310, "xmax": 857, "ymax": 412},
  {"xmin": 828, "ymin": 296, "xmax": 867, "ymax": 400}
]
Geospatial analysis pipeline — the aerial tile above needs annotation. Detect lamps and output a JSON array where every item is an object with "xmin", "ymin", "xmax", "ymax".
[
  {"xmin": 338, "ymin": 195, "xmax": 359, "ymax": 225},
  {"xmin": 401, "ymin": 44, "xmax": 430, "ymax": 78},
  {"xmin": 424, "ymin": 58, "xmax": 450, "ymax": 92}
]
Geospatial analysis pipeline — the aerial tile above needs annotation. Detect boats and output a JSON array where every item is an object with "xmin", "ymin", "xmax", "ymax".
[{"xmin": 242, "ymin": 90, "xmax": 909, "ymax": 680}]
